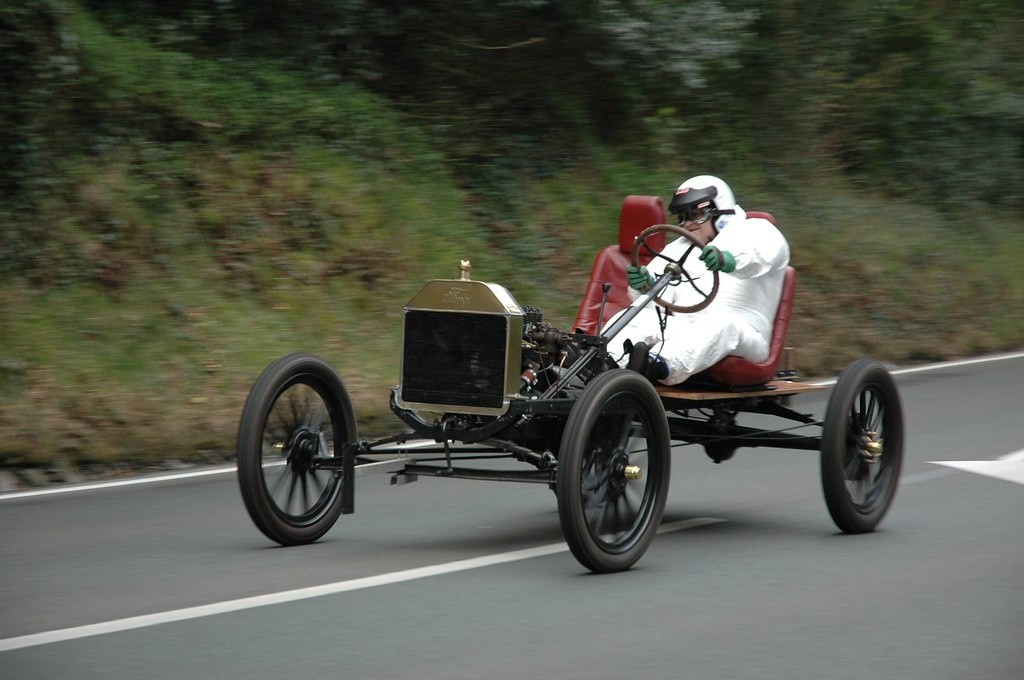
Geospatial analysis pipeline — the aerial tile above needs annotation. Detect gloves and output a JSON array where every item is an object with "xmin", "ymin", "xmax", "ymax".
[
  {"xmin": 626, "ymin": 264, "xmax": 654, "ymax": 294},
  {"xmin": 699, "ymin": 246, "xmax": 736, "ymax": 273}
]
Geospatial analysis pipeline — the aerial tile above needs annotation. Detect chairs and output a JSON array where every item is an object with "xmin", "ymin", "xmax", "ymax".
[
  {"xmin": 703, "ymin": 211, "xmax": 797, "ymax": 388},
  {"xmin": 571, "ymin": 195, "xmax": 667, "ymax": 346}
]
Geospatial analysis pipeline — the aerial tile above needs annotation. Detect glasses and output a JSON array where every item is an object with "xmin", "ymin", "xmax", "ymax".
[{"xmin": 677, "ymin": 207, "xmax": 716, "ymax": 225}]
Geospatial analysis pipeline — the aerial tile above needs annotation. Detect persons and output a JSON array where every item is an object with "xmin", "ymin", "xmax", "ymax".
[{"xmin": 558, "ymin": 175, "xmax": 790, "ymax": 384}]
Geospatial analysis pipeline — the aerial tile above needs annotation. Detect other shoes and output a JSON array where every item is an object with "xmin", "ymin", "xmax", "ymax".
[{"xmin": 626, "ymin": 342, "xmax": 668, "ymax": 380}]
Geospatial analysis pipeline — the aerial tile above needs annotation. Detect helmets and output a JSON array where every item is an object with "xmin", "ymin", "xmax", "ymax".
[{"xmin": 668, "ymin": 175, "xmax": 736, "ymax": 234}]
